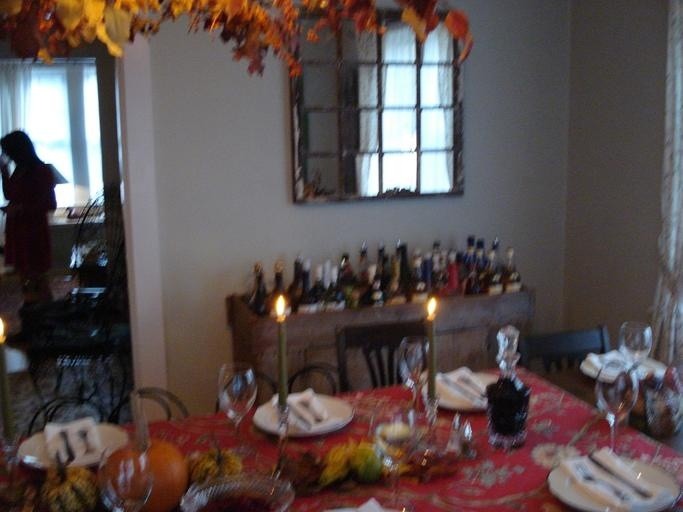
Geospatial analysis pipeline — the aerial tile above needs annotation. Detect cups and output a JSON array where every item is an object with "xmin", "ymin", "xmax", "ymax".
[{"xmin": 96, "ymin": 439, "xmax": 153, "ymax": 512}]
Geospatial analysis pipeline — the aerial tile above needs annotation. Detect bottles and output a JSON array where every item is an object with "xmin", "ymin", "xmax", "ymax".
[
  {"xmin": 246, "ymin": 235, "xmax": 522, "ymax": 318},
  {"xmin": 480, "ymin": 326, "xmax": 533, "ymax": 448}
]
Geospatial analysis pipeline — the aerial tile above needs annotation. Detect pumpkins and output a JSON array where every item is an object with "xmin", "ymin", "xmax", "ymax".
[
  {"xmin": 189, "ymin": 448, "xmax": 242, "ymax": 482},
  {"xmin": 37, "ymin": 467, "xmax": 100, "ymax": 512},
  {"xmin": 97, "ymin": 438, "xmax": 188, "ymax": 512}
]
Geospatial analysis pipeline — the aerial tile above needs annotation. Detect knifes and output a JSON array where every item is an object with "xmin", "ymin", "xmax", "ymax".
[{"xmin": 590, "ymin": 454, "xmax": 655, "ymax": 499}]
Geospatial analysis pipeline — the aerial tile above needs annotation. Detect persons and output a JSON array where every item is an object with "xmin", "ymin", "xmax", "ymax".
[{"xmin": 0, "ymin": 131, "xmax": 57, "ymax": 318}]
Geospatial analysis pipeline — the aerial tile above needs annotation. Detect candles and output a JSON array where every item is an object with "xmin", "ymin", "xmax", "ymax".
[
  {"xmin": 426, "ymin": 316, "xmax": 435, "ymax": 400},
  {"xmin": 276, "ymin": 315, "xmax": 288, "ymax": 408}
]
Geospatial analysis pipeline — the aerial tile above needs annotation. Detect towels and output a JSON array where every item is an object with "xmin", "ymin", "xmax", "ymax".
[
  {"xmin": 43, "ymin": 417, "xmax": 100, "ymax": 467},
  {"xmin": 562, "ymin": 446, "xmax": 676, "ymax": 511},
  {"xmin": 423, "ymin": 365, "xmax": 491, "ymax": 409},
  {"xmin": 271, "ymin": 386, "xmax": 328, "ymax": 432}
]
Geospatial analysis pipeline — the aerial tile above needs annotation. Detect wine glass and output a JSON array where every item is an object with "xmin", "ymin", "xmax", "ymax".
[
  {"xmin": 364, "ymin": 398, "xmax": 420, "ymax": 511},
  {"xmin": 592, "ymin": 320, "xmax": 653, "ymax": 459},
  {"xmin": 216, "ymin": 360, "xmax": 258, "ymax": 456},
  {"xmin": 393, "ymin": 333, "xmax": 430, "ymax": 417}
]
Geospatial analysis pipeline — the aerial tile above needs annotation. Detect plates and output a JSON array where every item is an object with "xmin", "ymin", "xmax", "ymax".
[
  {"xmin": 16, "ymin": 422, "xmax": 128, "ymax": 471},
  {"xmin": 547, "ymin": 454, "xmax": 683, "ymax": 512},
  {"xmin": 419, "ymin": 370, "xmax": 500, "ymax": 413},
  {"xmin": 252, "ymin": 393, "xmax": 355, "ymax": 438}
]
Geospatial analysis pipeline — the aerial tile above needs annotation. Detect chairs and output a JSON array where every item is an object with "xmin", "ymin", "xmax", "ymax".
[
  {"xmin": 17, "ymin": 190, "xmax": 128, "ymax": 405},
  {"xmin": 336, "ymin": 319, "xmax": 430, "ymax": 392},
  {"xmin": 487, "ymin": 322, "xmax": 611, "ymax": 377}
]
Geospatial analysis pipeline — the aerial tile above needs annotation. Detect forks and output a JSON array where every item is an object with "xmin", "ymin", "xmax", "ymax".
[{"xmin": 575, "ymin": 460, "xmax": 632, "ymax": 502}]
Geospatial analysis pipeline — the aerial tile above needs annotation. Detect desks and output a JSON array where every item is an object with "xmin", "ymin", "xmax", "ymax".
[{"xmin": 227, "ymin": 286, "xmax": 536, "ymax": 409}]
